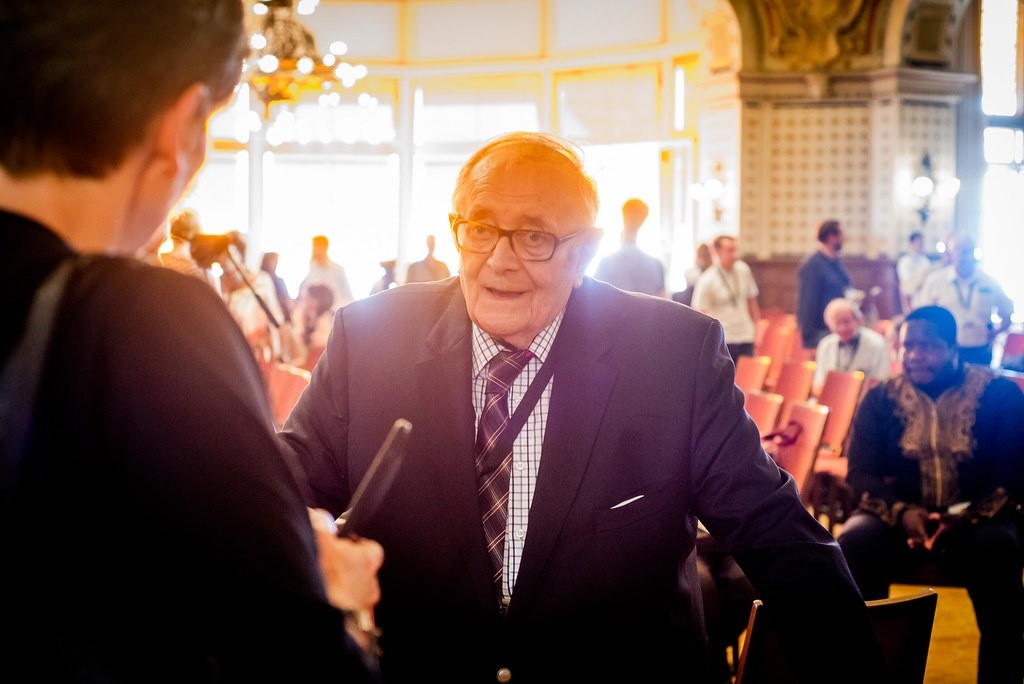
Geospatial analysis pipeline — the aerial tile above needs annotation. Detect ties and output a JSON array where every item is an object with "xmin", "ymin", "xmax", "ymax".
[
  {"xmin": 452, "ymin": 213, "xmax": 586, "ymax": 262},
  {"xmin": 475, "ymin": 350, "xmax": 536, "ymax": 615}
]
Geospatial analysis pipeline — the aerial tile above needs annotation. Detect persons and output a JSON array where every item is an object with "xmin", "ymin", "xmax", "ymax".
[
  {"xmin": 1, "ymin": 1, "xmax": 385, "ymax": 684},
  {"xmin": 276, "ymin": 131, "xmax": 890, "ymax": 684},
  {"xmin": 921, "ymin": 237, "xmax": 1014, "ymax": 364},
  {"xmin": 836, "ymin": 305, "xmax": 1024, "ymax": 684},
  {"xmin": 796, "ymin": 217, "xmax": 852, "ymax": 348},
  {"xmin": 811, "ymin": 299, "xmax": 890, "ymax": 396},
  {"xmin": 894, "ymin": 231, "xmax": 951, "ymax": 316},
  {"xmin": 138, "ymin": 199, "xmax": 765, "ymax": 367}
]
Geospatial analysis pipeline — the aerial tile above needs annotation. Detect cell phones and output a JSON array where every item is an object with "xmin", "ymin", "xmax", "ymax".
[{"xmin": 335, "ymin": 420, "xmax": 413, "ymax": 541}]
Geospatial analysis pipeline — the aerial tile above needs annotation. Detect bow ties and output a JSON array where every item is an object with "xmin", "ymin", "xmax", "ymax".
[{"xmin": 839, "ymin": 339, "xmax": 857, "ymax": 346}]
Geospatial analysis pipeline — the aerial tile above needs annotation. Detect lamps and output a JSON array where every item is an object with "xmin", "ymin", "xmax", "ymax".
[{"xmin": 239, "ymin": 0, "xmax": 366, "ymax": 121}]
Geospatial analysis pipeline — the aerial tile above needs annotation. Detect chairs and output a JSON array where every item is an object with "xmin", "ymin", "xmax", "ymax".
[{"xmin": 685, "ymin": 311, "xmax": 900, "ymax": 675}]
